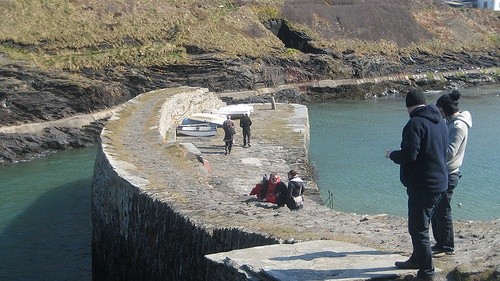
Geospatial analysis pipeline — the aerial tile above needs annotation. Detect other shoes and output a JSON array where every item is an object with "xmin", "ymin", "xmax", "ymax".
[
  {"xmin": 431, "ymin": 246, "xmax": 455, "ymax": 257},
  {"xmin": 395, "ymin": 257, "xmax": 420, "ymax": 269},
  {"xmin": 404, "ymin": 273, "xmax": 434, "ymax": 281}
]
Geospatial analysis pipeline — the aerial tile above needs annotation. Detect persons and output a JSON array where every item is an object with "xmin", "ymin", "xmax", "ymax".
[
  {"xmin": 387, "ymin": 89, "xmax": 453, "ymax": 281},
  {"xmin": 250, "ymin": 170, "xmax": 305, "ymax": 209},
  {"xmin": 223, "ymin": 116, "xmax": 236, "ymax": 155},
  {"xmin": 240, "ymin": 114, "xmax": 252, "ymax": 147},
  {"xmin": 428, "ymin": 91, "xmax": 474, "ymax": 257}
]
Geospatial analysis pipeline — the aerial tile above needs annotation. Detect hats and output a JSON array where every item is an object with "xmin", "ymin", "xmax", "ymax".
[
  {"xmin": 406, "ymin": 89, "xmax": 427, "ymax": 107},
  {"xmin": 436, "ymin": 90, "xmax": 461, "ymax": 112}
]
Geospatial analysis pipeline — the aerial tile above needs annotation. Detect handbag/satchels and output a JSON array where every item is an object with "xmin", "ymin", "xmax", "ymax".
[{"xmin": 294, "ymin": 196, "xmax": 303, "ymax": 207}]
